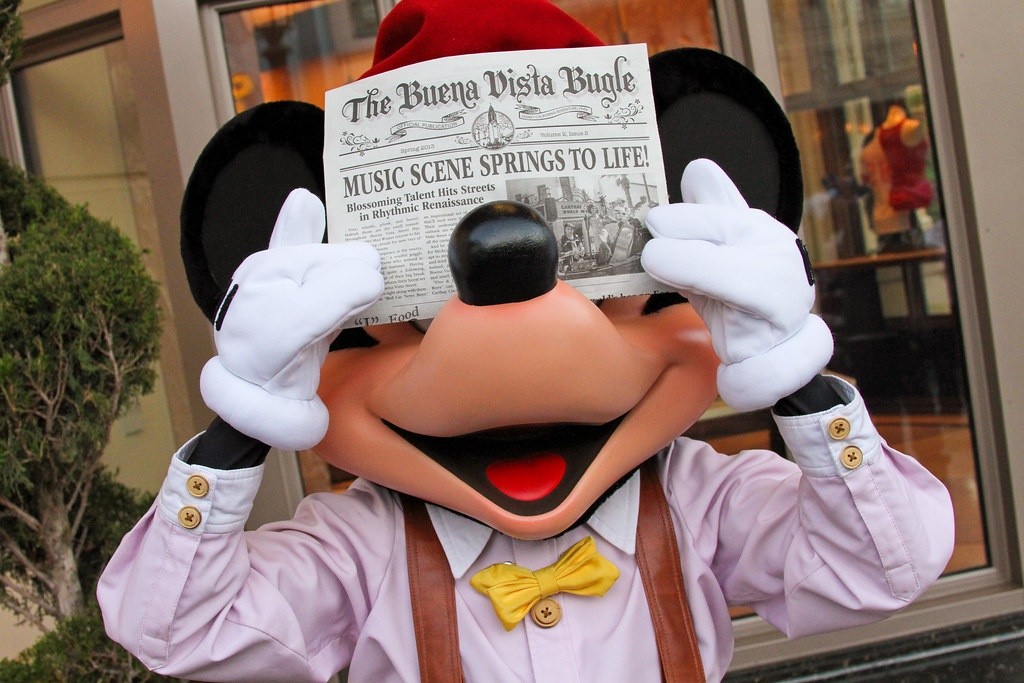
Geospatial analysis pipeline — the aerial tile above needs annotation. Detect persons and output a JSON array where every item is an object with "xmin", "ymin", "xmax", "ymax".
[{"xmin": 874, "ymin": 100, "xmax": 932, "ymax": 209}]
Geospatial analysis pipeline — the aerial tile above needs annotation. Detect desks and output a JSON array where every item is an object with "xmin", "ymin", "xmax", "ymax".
[{"xmin": 815, "ymin": 247, "xmax": 967, "ymax": 415}]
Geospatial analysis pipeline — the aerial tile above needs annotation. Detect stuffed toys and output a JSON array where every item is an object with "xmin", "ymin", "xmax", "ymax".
[{"xmin": 97, "ymin": 0, "xmax": 956, "ymax": 683}]
